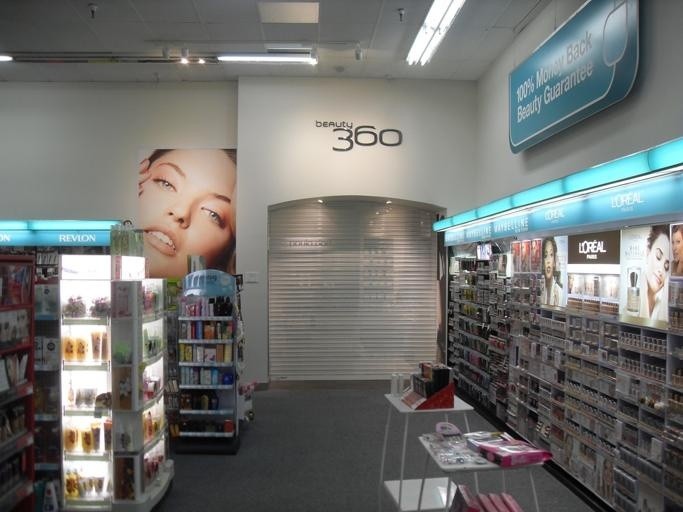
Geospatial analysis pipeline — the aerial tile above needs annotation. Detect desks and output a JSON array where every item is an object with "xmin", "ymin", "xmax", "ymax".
[
  {"xmin": 416, "ymin": 432, "xmax": 544, "ymax": 512},
  {"xmin": 377, "ymin": 392, "xmax": 480, "ymax": 512}
]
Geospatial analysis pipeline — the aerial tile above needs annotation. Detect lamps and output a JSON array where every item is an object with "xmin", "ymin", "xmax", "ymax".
[
  {"xmin": 182, "ymin": 48, "xmax": 190, "ymax": 59},
  {"xmin": 216, "ymin": 50, "xmax": 319, "ymax": 67},
  {"xmin": 354, "ymin": 46, "xmax": 362, "ymax": 61},
  {"xmin": 162, "ymin": 48, "xmax": 171, "ymax": 59},
  {"xmin": 406, "ymin": 0, "xmax": 467, "ymax": 67}
]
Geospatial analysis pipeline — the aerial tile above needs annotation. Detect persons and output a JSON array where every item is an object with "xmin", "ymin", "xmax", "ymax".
[
  {"xmin": 541, "ymin": 236, "xmax": 563, "ymax": 307},
  {"xmin": 671, "ymin": 225, "xmax": 683, "ymax": 276},
  {"xmin": 477, "ymin": 245, "xmax": 483, "ymax": 260},
  {"xmin": 138, "ymin": 148, "xmax": 238, "ymax": 280},
  {"xmin": 638, "ymin": 224, "xmax": 669, "ymax": 321}
]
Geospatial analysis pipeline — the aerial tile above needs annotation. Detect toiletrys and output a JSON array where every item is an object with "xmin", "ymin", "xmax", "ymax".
[
  {"xmin": 626, "ymin": 266, "xmax": 642, "ymax": 317},
  {"xmin": 0, "ymin": 292, "xmax": 235, "ymax": 512}
]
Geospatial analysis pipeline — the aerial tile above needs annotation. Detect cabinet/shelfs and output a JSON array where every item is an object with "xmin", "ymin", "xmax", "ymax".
[{"xmin": 433, "ymin": 135, "xmax": 683, "ymax": 511}]
